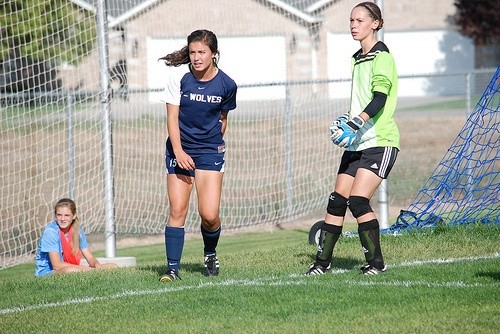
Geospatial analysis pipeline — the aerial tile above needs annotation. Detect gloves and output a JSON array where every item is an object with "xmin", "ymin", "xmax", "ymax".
[
  {"xmin": 330, "ymin": 112, "xmax": 350, "ymax": 134},
  {"xmin": 330, "ymin": 115, "xmax": 366, "ymax": 148}
]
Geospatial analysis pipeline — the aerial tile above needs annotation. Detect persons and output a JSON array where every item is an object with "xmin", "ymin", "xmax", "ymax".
[
  {"xmin": 298, "ymin": 1, "xmax": 400, "ymax": 276},
  {"xmin": 156, "ymin": 29, "xmax": 238, "ymax": 281},
  {"xmin": 33, "ymin": 198, "xmax": 119, "ymax": 277}
]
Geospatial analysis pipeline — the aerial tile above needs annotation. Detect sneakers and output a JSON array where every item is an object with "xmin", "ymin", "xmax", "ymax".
[
  {"xmin": 360, "ymin": 264, "xmax": 387, "ymax": 276},
  {"xmin": 305, "ymin": 261, "xmax": 332, "ymax": 276},
  {"xmin": 204, "ymin": 247, "xmax": 220, "ymax": 277},
  {"xmin": 159, "ymin": 269, "xmax": 181, "ymax": 283}
]
